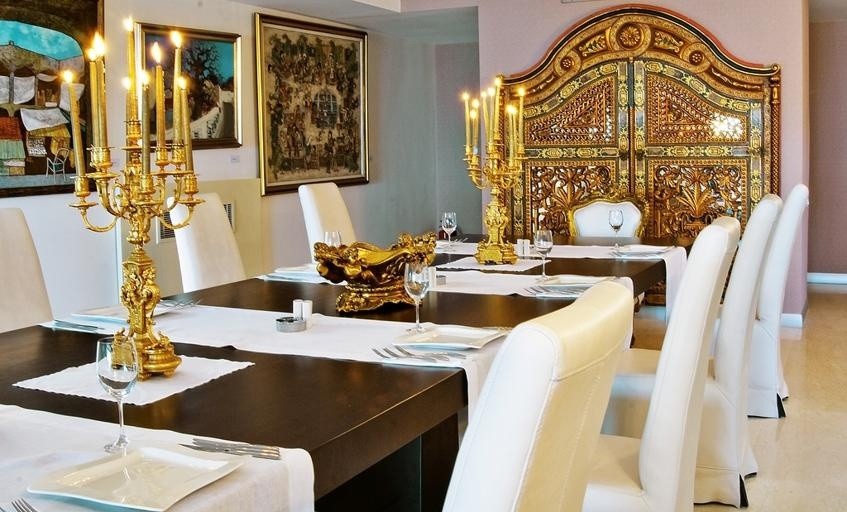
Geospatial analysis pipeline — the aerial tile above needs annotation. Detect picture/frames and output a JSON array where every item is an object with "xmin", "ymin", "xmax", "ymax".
[
  {"xmin": 0, "ymin": 0, "xmax": 113, "ymax": 199},
  {"xmin": 135, "ymin": 17, "xmax": 244, "ymax": 153},
  {"xmin": 253, "ymin": 10, "xmax": 371, "ymax": 198}
]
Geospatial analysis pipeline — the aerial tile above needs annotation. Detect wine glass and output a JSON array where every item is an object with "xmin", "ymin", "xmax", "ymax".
[
  {"xmin": 534, "ymin": 228, "xmax": 552, "ymax": 281},
  {"xmin": 96, "ymin": 336, "xmax": 137, "ymax": 451},
  {"xmin": 608, "ymin": 209, "xmax": 623, "ymax": 250},
  {"xmin": 440, "ymin": 211, "xmax": 457, "ymax": 253},
  {"xmin": 403, "ymin": 262, "xmax": 429, "ymax": 322}
]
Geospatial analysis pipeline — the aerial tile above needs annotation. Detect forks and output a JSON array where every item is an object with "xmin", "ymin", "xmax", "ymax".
[
  {"xmin": 523, "ymin": 284, "xmax": 584, "ymax": 297},
  {"xmin": 370, "ymin": 344, "xmax": 467, "ymax": 363},
  {"xmin": 158, "ymin": 298, "xmax": 200, "ymax": 311},
  {"xmin": 443, "ymin": 237, "xmax": 469, "ymax": 247},
  {"xmin": 10, "ymin": 497, "xmax": 41, "ymax": 512},
  {"xmin": 609, "ymin": 251, "xmax": 658, "ymax": 258}
]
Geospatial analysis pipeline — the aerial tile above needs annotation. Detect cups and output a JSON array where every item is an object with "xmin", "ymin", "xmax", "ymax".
[{"xmin": 323, "ymin": 231, "xmax": 342, "ymax": 247}]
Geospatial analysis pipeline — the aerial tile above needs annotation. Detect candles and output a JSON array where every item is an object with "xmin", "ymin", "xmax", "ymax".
[
  {"xmin": 459, "ymin": 76, "xmax": 527, "ymax": 170},
  {"xmin": 58, "ymin": 14, "xmax": 195, "ymax": 178}
]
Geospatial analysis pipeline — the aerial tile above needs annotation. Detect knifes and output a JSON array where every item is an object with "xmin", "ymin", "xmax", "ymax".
[
  {"xmin": 177, "ymin": 436, "xmax": 281, "ymax": 460},
  {"xmin": 54, "ymin": 319, "xmax": 106, "ymax": 332}
]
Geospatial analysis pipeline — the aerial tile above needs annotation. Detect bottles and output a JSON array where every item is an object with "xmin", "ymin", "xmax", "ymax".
[
  {"xmin": 428, "ymin": 267, "xmax": 437, "ymax": 288},
  {"xmin": 292, "ymin": 298, "xmax": 311, "ymax": 320},
  {"xmin": 517, "ymin": 239, "xmax": 529, "ymax": 253}
]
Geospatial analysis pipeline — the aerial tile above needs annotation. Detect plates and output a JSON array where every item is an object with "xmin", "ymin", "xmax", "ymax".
[
  {"xmin": 71, "ymin": 302, "xmax": 175, "ymax": 321},
  {"xmin": 532, "ymin": 274, "xmax": 615, "ymax": 286},
  {"xmin": 393, "ymin": 324, "xmax": 505, "ymax": 349},
  {"xmin": 274, "ymin": 263, "xmax": 321, "ymax": 277},
  {"xmin": 26, "ymin": 446, "xmax": 248, "ymax": 510},
  {"xmin": 617, "ymin": 243, "xmax": 674, "ymax": 254}
]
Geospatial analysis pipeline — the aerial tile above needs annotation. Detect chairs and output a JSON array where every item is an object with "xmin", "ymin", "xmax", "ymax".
[
  {"xmin": 706, "ymin": 182, "xmax": 810, "ymax": 421},
  {"xmin": 581, "ymin": 214, "xmax": 743, "ymax": 512},
  {"xmin": 0, "ymin": 205, "xmax": 54, "ymax": 335},
  {"xmin": 163, "ymin": 190, "xmax": 249, "ymax": 295},
  {"xmin": 297, "ymin": 179, "xmax": 359, "ymax": 265},
  {"xmin": 614, "ymin": 191, "xmax": 785, "ymax": 511},
  {"xmin": 439, "ymin": 280, "xmax": 638, "ymax": 511},
  {"xmin": 564, "ymin": 191, "xmax": 653, "ymax": 247}
]
